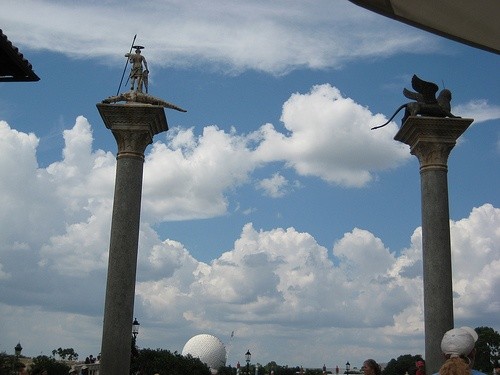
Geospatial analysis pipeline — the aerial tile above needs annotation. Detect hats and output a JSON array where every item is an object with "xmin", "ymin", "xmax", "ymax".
[{"xmin": 441, "ymin": 327, "xmax": 479, "ymax": 356}]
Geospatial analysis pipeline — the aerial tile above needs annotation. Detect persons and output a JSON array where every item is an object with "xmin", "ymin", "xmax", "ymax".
[
  {"xmin": 125, "ymin": 48, "xmax": 149, "ymax": 93},
  {"xmin": 431, "ymin": 326, "xmax": 489, "ymax": 375},
  {"xmin": 130, "ymin": 367, "xmax": 161, "ymax": 375},
  {"xmin": 492, "ymin": 364, "xmax": 500, "ymax": 375},
  {"xmin": 323, "ymin": 359, "xmax": 381, "ymax": 375},
  {"xmin": 403, "ymin": 358, "xmax": 426, "ymax": 375},
  {"xmin": 199, "ymin": 367, "xmax": 301, "ymax": 375},
  {"xmin": 68, "ymin": 368, "xmax": 78, "ymax": 375},
  {"xmin": 32, "ymin": 368, "xmax": 47, "ymax": 375},
  {"xmin": 84, "ymin": 354, "xmax": 101, "ymax": 375}
]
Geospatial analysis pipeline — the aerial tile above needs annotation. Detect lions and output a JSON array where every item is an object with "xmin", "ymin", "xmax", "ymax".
[{"xmin": 371, "ymin": 74, "xmax": 466, "ymax": 132}]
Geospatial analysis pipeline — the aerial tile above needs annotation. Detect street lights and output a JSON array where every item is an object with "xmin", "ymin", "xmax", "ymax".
[
  {"xmin": 132, "ymin": 318, "xmax": 141, "ymax": 346},
  {"xmin": 245, "ymin": 349, "xmax": 252, "ymax": 375},
  {"xmin": 345, "ymin": 361, "xmax": 351, "ymax": 375}
]
